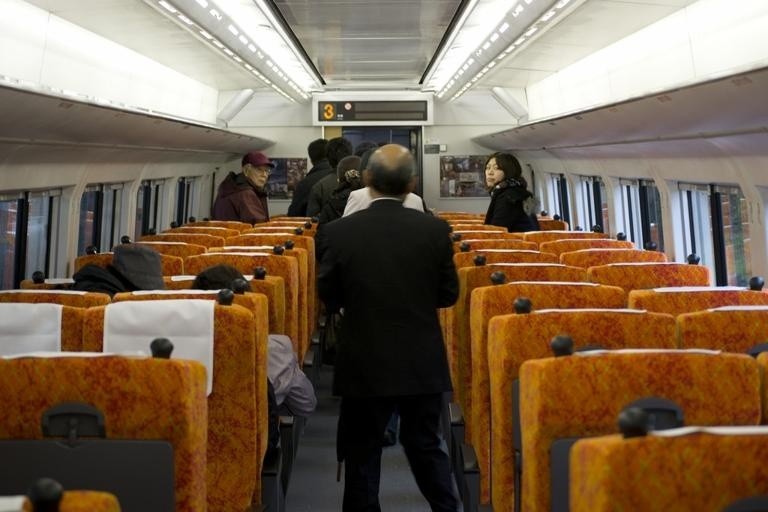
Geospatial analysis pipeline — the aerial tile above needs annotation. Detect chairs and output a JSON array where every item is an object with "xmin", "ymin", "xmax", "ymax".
[
  {"xmin": 445, "ymin": 262, "xmax": 767, "ymax": 509},
  {"xmin": 2, "ymin": 359, "xmax": 205, "ymax": 510},
  {"xmin": 83, "ymin": 291, "xmax": 269, "ymax": 512},
  {"xmin": 436, "ymin": 207, "xmax": 667, "ymax": 262},
  {"xmin": 21, "ymin": 216, "xmax": 320, "ymax": 360},
  {"xmin": 0, "ymin": 301, "xmax": 90, "ymax": 356}
]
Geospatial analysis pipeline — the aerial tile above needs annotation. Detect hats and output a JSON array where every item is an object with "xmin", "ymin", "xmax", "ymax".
[
  {"xmin": 113, "ymin": 243, "xmax": 166, "ymax": 287},
  {"xmin": 241, "ymin": 151, "xmax": 276, "ymax": 170}
]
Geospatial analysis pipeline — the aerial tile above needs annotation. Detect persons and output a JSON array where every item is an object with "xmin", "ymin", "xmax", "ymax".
[
  {"xmin": 319, "ymin": 154, "xmax": 366, "ymax": 219},
  {"xmin": 344, "ymin": 148, "xmax": 428, "ymax": 215},
  {"xmin": 189, "ymin": 266, "xmax": 318, "ymax": 418},
  {"xmin": 213, "ymin": 152, "xmax": 272, "ymax": 226},
  {"xmin": 317, "ymin": 143, "xmax": 461, "ymax": 512},
  {"xmin": 286, "ymin": 140, "xmax": 327, "ymax": 216},
  {"xmin": 307, "ymin": 138, "xmax": 353, "ymax": 218},
  {"xmin": 74, "ymin": 245, "xmax": 164, "ymax": 293},
  {"xmin": 483, "ymin": 152, "xmax": 538, "ymax": 235}
]
